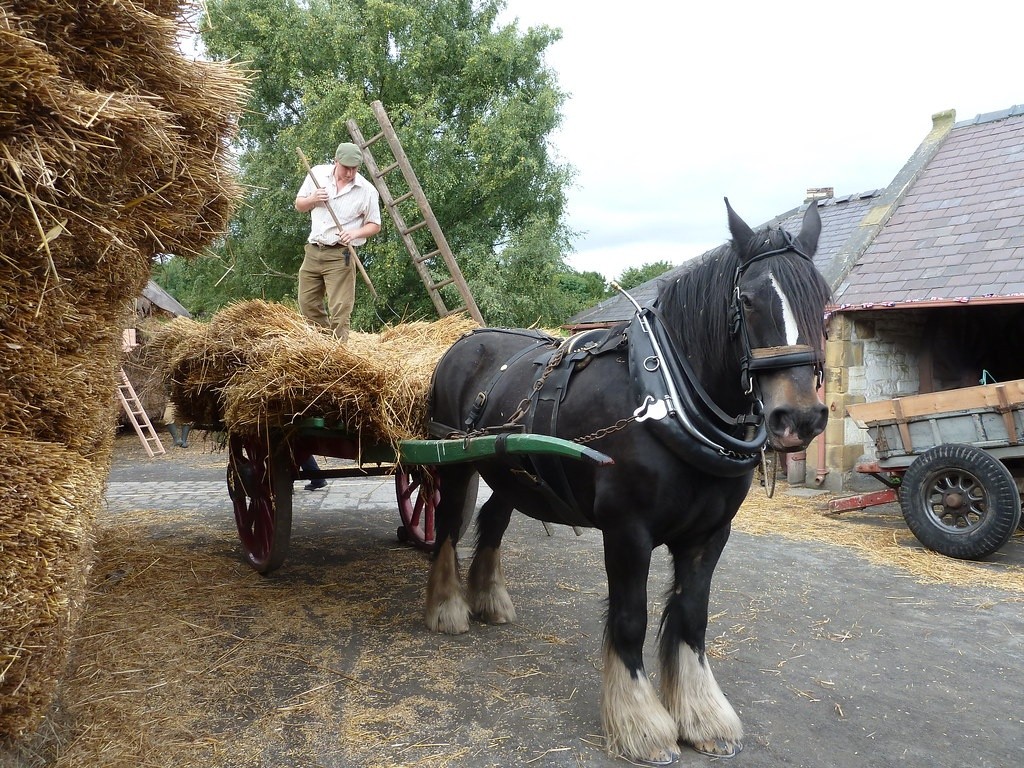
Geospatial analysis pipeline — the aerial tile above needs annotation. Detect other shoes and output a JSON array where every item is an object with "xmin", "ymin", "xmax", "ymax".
[{"xmin": 304, "ymin": 479, "xmax": 328, "ymax": 490}]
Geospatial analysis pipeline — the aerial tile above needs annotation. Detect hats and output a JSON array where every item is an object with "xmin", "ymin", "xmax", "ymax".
[{"xmin": 334, "ymin": 142, "xmax": 363, "ymax": 167}]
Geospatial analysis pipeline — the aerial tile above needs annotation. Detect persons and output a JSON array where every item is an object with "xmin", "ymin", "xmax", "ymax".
[
  {"xmin": 163, "ymin": 393, "xmax": 192, "ymax": 447},
  {"xmin": 296, "ymin": 143, "xmax": 381, "ymax": 340},
  {"xmin": 301, "ymin": 455, "xmax": 327, "ymax": 490}
]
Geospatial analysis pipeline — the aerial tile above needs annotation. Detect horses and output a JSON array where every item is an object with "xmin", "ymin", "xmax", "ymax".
[{"xmin": 425, "ymin": 196, "xmax": 832, "ymax": 765}]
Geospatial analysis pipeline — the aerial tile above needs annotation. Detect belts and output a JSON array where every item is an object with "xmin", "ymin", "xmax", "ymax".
[{"xmin": 311, "ymin": 242, "xmax": 344, "ymax": 249}]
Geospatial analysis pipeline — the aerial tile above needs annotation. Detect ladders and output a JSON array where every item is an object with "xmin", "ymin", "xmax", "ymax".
[
  {"xmin": 116, "ymin": 367, "xmax": 166, "ymax": 459},
  {"xmin": 346, "ymin": 99, "xmax": 487, "ymax": 329}
]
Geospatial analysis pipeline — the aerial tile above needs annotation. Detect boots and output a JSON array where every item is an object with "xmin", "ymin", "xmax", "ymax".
[
  {"xmin": 165, "ymin": 422, "xmax": 183, "ymax": 446},
  {"xmin": 180, "ymin": 425, "xmax": 191, "ymax": 449}
]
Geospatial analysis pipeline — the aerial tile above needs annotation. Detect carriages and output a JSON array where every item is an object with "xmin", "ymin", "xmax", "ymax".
[{"xmin": 193, "ymin": 195, "xmax": 833, "ymax": 768}]
